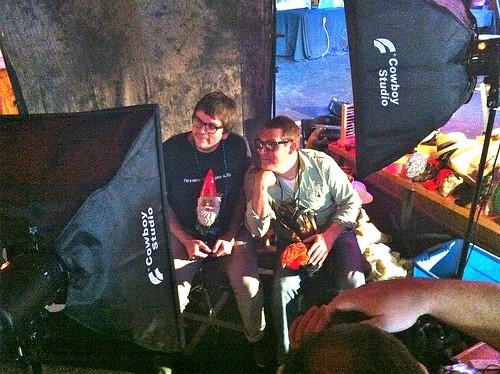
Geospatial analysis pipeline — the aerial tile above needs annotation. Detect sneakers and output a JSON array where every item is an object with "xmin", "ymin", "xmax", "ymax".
[{"xmin": 251, "ymin": 341, "xmax": 272, "ymax": 367}]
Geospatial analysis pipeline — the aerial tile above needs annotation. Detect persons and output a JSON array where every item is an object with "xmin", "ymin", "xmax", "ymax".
[
  {"xmin": 196, "ymin": 168, "xmax": 222, "ymax": 226},
  {"xmin": 162, "ymin": 91, "xmax": 274, "ymax": 367},
  {"xmin": 243, "ymin": 115, "xmax": 367, "ymax": 374},
  {"xmin": 282, "ymin": 277, "xmax": 500, "ymax": 374}
]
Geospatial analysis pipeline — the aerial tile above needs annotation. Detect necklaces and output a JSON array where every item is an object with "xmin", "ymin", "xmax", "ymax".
[{"xmin": 191, "ymin": 129, "xmax": 228, "ymax": 226}]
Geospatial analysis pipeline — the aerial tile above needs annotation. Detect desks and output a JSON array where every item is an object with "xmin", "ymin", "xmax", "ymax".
[{"xmin": 328, "ymin": 135, "xmax": 500, "ymax": 257}]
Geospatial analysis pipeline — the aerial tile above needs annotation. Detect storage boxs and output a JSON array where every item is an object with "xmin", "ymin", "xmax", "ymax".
[{"xmin": 412, "ymin": 239, "xmax": 500, "ymax": 282}]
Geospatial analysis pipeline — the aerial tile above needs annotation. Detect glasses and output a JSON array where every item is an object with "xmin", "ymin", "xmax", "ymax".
[
  {"xmin": 192, "ymin": 114, "xmax": 224, "ymax": 134},
  {"xmin": 255, "ymin": 138, "xmax": 292, "ymax": 151}
]
